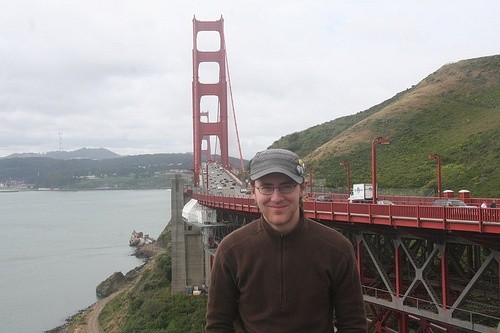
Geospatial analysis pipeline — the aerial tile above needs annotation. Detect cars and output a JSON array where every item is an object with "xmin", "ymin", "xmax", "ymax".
[
  {"xmin": 432, "ymin": 198, "xmax": 466, "ymax": 207},
  {"xmin": 203, "ymin": 165, "xmax": 252, "ymax": 195},
  {"xmin": 316, "ymin": 195, "xmax": 333, "ymax": 202},
  {"xmin": 376, "ymin": 200, "xmax": 394, "ymax": 204}
]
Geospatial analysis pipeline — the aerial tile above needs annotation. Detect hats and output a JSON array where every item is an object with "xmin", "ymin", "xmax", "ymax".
[{"xmin": 250, "ymin": 148, "xmax": 306, "ymax": 183}]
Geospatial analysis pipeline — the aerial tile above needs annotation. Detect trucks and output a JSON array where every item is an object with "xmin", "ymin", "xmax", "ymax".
[{"xmin": 347, "ymin": 183, "xmax": 379, "ymax": 203}]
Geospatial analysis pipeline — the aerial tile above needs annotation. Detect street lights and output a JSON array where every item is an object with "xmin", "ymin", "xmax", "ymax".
[
  {"xmin": 339, "ymin": 160, "xmax": 351, "ymax": 193},
  {"xmin": 372, "ymin": 136, "xmax": 393, "ymax": 204},
  {"xmin": 427, "ymin": 153, "xmax": 440, "ymax": 200},
  {"xmin": 206, "ymin": 159, "xmax": 212, "ymax": 196}
]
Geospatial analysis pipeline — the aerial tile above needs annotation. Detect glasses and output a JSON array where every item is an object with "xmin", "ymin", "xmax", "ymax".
[{"xmin": 254, "ymin": 183, "xmax": 298, "ymax": 194}]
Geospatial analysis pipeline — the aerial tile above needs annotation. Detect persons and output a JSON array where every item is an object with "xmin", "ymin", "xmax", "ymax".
[{"xmin": 205, "ymin": 149, "xmax": 369, "ymax": 333}]
